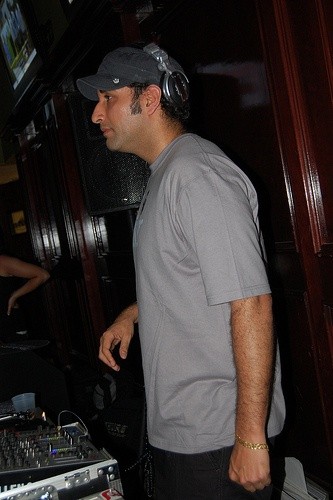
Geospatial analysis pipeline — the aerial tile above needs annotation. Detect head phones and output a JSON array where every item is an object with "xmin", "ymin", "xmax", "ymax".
[{"xmin": 129, "ymin": 38, "xmax": 191, "ymax": 115}]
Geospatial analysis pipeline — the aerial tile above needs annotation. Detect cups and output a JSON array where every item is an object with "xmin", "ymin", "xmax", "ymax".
[{"xmin": 12, "ymin": 393, "xmax": 35, "ymax": 412}]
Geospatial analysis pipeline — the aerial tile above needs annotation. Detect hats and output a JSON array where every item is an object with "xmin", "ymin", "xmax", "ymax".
[{"xmin": 76, "ymin": 47, "xmax": 185, "ymax": 100}]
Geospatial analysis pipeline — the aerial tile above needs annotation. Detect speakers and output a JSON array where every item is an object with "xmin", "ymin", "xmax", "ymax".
[{"xmin": 67, "ymin": 91, "xmax": 151, "ymax": 217}]
